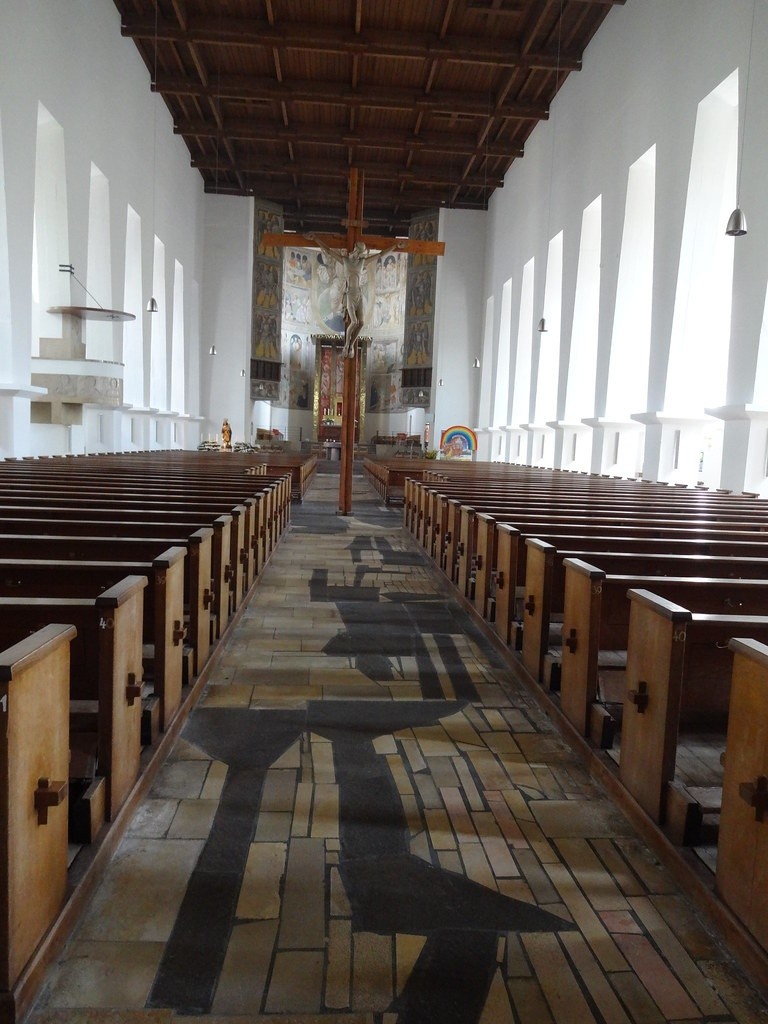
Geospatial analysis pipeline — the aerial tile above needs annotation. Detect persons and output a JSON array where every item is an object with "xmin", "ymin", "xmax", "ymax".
[
  {"xmin": 256, "ymin": 263, "xmax": 279, "ymax": 308},
  {"xmin": 411, "ymin": 272, "xmax": 433, "ymax": 314},
  {"xmin": 256, "ymin": 210, "xmax": 279, "ymax": 256},
  {"xmin": 256, "ymin": 314, "xmax": 276, "ymax": 359},
  {"xmin": 222, "ymin": 420, "xmax": 231, "ymax": 448},
  {"xmin": 302, "ymin": 232, "xmax": 405, "ymax": 359},
  {"xmin": 288, "ymin": 251, "xmax": 311, "ymax": 281},
  {"xmin": 409, "ymin": 320, "xmax": 428, "ymax": 364},
  {"xmin": 411, "ymin": 221, "xmax": 435, "ymax": 264}
]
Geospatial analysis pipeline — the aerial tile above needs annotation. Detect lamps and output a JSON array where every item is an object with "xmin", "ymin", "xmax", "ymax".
[
  {"xmin": 537, "ymin": 317, "xmax": 548, "ymax": 331},
  {"xmin": 438, "ymin": 378, "xmax": 444, "ymax": 386},
  {"xmin": 209, "ymin": 344, "xmax": 217, "ymax": 355},
  {"xmin": 472, "ymin": 357, "xmax": 480, "ymax": 368},
  {"xmin": 419, "ymin": 391, "xmax": 424, "ymax": 397},
  {"xmin": 240, "ymin": 369, "xmax": 246, "ymax": 377},
  {"xmin": 724, "ymin": 204, "xmax": 747, "ymax": 237},
  {"xmin": 146, "ymin": 298, "xmax": 159, "ymax": 311},
  {"xmin": 259, "ymin": 383, "xmax": 263, "ymax": 390}
]
